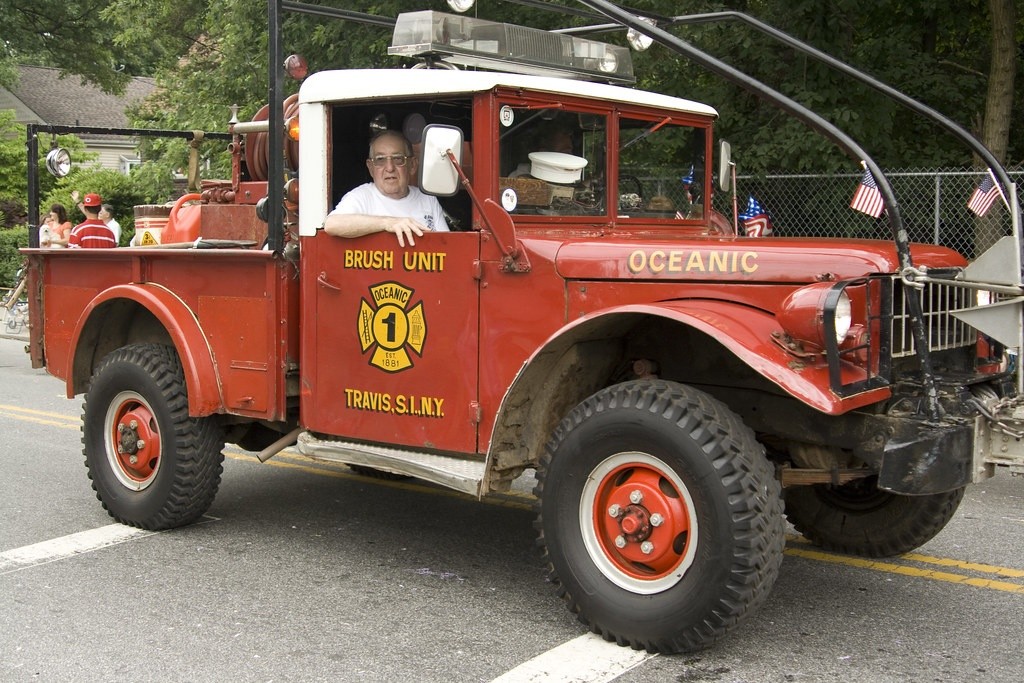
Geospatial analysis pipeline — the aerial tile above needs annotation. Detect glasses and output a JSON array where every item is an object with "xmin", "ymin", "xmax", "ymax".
[{"xmin": 369, "ymin": 155, "xmax": 413, "ymax": 167}]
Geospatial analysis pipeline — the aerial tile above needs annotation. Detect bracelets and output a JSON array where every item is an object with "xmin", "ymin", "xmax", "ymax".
[{"xmin": 76, "ymin": 201, "xmax": 81, "ymax": 206}]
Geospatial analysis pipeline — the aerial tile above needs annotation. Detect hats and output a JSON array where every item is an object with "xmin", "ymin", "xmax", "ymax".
[
  {"xmin": 81, "ymin": 192, "xmax": 102, "ymax": 206},
  {"xmin": 528, "ymin": 151, "xmax": 589, "ymax": 185}
]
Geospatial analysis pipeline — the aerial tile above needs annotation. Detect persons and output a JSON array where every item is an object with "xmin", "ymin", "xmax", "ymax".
[
  {"xmin": 324, "ymin": 131, "xmax": 450, "ymax": 247},
  {"xmin": 507, "ymin": 126, "xmax": 573, "ymax": 179},
  {"xmin": 39, "ymin": 190, "xmax": 122, "ymax": 249}
]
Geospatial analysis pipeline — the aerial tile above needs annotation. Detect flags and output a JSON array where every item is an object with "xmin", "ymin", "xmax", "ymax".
[
  {"xmin": 967, "ymin": 174, "xmax": 1002, "ymax": 217},
  {"xmin": 850, "ymin": 166, "xmax": 885, "ymax": 218},
  {"xmin": 681, "ymin": 164, "xmax": 694, "ymax": 201},
  {"xmin": 738, "ymin": 196, "xmax": 772, "ymax": 237}
]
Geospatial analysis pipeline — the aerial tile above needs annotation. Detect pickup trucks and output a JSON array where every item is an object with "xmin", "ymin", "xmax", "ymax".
[{"xmin": 17, "ymin": 0, "xmax": 1024, "ymax": 658}]
